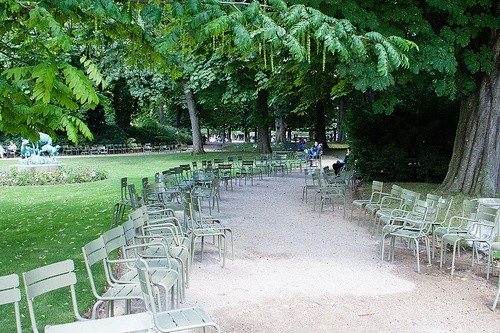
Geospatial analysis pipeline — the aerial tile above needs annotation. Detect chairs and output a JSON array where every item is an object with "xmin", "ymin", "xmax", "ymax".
[{"xmin": 0, "ymin": 143, "xmax": 500, "ymax": 333}]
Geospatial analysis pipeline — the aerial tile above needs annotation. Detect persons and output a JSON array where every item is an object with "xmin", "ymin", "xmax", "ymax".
[
  {"xmin": 298, "ymin": 138, "xmax": 323, "ymax": 161},
  {"xmin": 0, "ymin": 140, "xmax": 17, "ymax": 159},
  {"xmin": 332, "ymin": 147, "xmax": 351, "ymax": 177}
]
